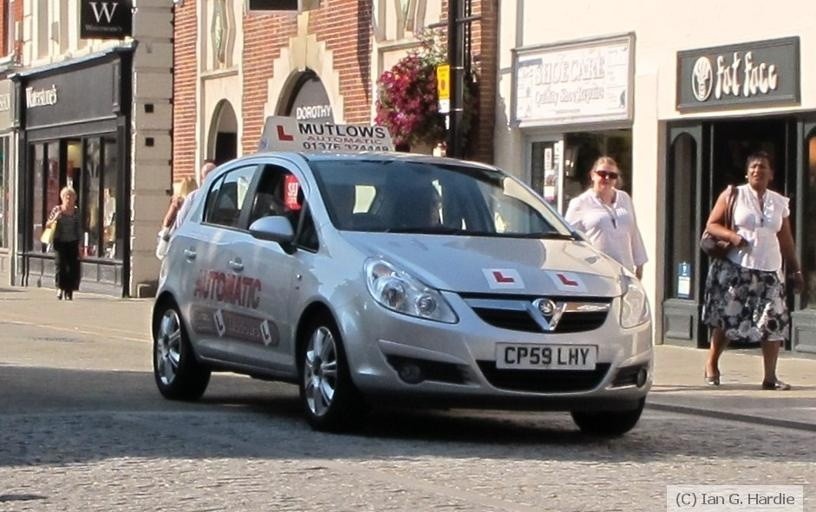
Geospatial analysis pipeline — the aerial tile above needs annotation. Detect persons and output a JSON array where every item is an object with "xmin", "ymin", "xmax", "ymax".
[
  {"xmin": 177, "ymin": 160, "xmax": 217, "ymax": 230},
  {"xmin": 562, "ymin": 155, "xmax": 648, "ymax": 281},
  {"xmin": 46, "ymin": 186, "xmax": 82, "ymax": 300},
  {"xmin": 383, "ymin": 181, "xmax": 440, "ymax": 235},
  {"xmin": 700, "ymin": 150, "xmax": 804, "ymax": 391},
  {"xmin": 162, "ymin": 176, "xmax": 200, "ymax": 240}
]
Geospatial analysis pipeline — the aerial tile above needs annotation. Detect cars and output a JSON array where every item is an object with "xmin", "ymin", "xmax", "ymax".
[{"xmin": 152, "ymin": 117, "xmax": 652, "ymax": 437}]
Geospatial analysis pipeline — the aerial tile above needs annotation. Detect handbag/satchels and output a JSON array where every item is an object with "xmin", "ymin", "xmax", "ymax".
[
  {"xmin": 698, "ymin": 226, "xmax": 732, "ymax": 256},
  {"xmin": 40, "ymin": 221, "xmax": 58, "ymax": 244}
]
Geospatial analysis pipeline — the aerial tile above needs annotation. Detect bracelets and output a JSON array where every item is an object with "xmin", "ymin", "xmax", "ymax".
[{"xmin": 791, "ymin": 270, "xmax": 802, "ymax": 275}]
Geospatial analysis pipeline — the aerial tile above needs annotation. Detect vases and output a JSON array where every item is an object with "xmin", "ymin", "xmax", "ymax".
[{"xmin": 409, "ymin": 136, "xmax": 434, "ymax": 156}]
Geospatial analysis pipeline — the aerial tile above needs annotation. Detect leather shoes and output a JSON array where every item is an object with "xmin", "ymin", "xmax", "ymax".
[
  {"xmin": 704, "ymin": 364, "xmax": 719, "ymax": 385},
  {"xmin": 57, "ymin": 288, "xmax": 72, "ymax": 300},
  {"xmin": 763, "ymin": 380, "xmax": 790, "ymax": 390}
]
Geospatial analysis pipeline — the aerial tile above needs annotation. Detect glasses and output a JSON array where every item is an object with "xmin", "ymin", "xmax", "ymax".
[{"xmin": 594, "ymin": 170, "xmax": 618, "ymax": 179}]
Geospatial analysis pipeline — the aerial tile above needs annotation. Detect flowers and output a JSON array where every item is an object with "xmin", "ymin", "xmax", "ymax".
[{"xmin": 368, "ymin": 50, "xmax": 450, "ymax": 146}]
